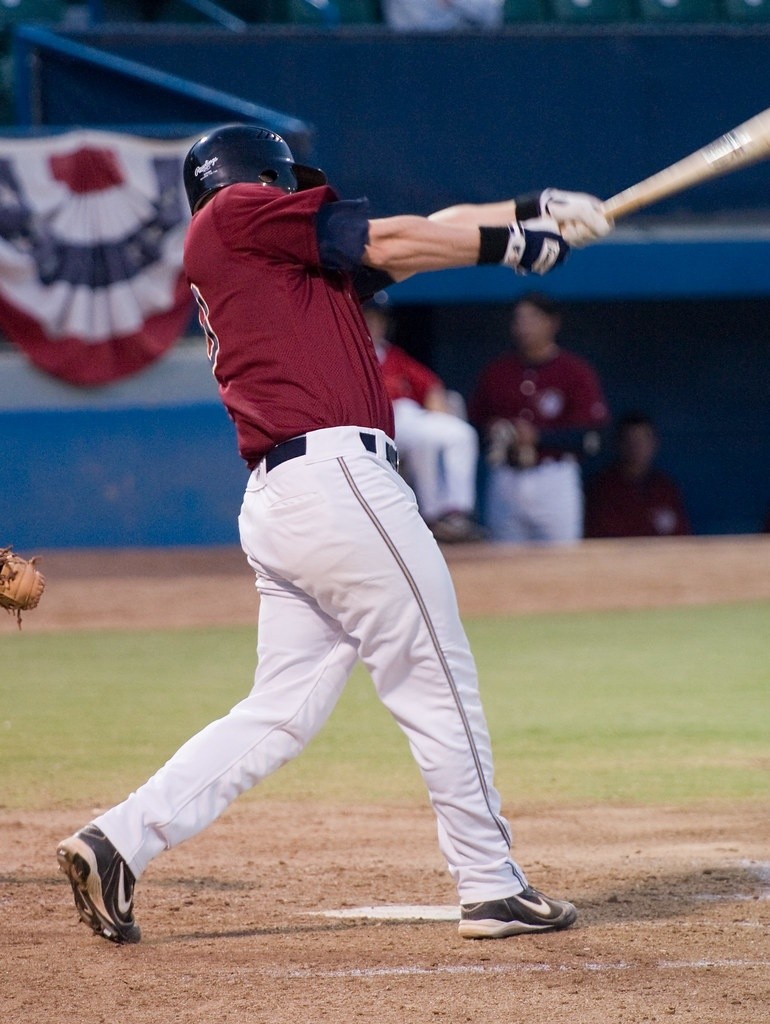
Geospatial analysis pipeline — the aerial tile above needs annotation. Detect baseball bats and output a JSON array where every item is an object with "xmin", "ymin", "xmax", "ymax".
[{"xmin": 559, "ymin": 107, "xmax": 770, "ymax": 234}]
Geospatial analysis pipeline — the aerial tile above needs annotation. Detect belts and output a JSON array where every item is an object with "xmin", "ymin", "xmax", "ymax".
[{"xmin": 265, "ymin": 432, "xmax": 401, "ymax": 473}]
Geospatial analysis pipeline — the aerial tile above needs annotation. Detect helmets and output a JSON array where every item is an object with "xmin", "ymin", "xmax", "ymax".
[{"xmin": 183, "ymin": 124, "xmax": 327, "ymax": 216}]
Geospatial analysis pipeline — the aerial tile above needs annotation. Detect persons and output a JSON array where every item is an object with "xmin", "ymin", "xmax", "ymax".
[
  {"xmin": 362, "ymin": 291, "xmax": 681, "ymax": 542},
  {"xmin": 53, "ymin": 125, "xmax": 614, "ymax": 944}
]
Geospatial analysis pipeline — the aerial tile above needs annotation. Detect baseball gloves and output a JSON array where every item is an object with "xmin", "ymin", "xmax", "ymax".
[{"xmin": 0, "ymin": 548, "xmax": 47, "ymax": 611}]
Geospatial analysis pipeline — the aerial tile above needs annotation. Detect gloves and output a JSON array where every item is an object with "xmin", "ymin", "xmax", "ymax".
[
  {"xmin": 486, "ymin": 417, "xmax": 519, "ymax": 466},
  {"xmin": 500, "ymin": 216, "xmax": 571, "ymax": 278},
  {"xmin": 535, "ymin": 186, "xmax": 615, "ymax": 249}
]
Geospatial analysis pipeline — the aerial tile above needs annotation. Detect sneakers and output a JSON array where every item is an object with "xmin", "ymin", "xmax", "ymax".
[
  {"xmin": 55, "ymin": 823, "xmax": 141, "ymax": 944},
  {"xmin": 458, "ymin": 884, "xmax": 578, "ymax": 938}
]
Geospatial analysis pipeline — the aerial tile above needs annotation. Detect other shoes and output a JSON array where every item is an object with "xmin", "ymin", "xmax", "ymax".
[{"xmin": 428, "ymin": 513, "xmax": 479, "ymax": 542}]
